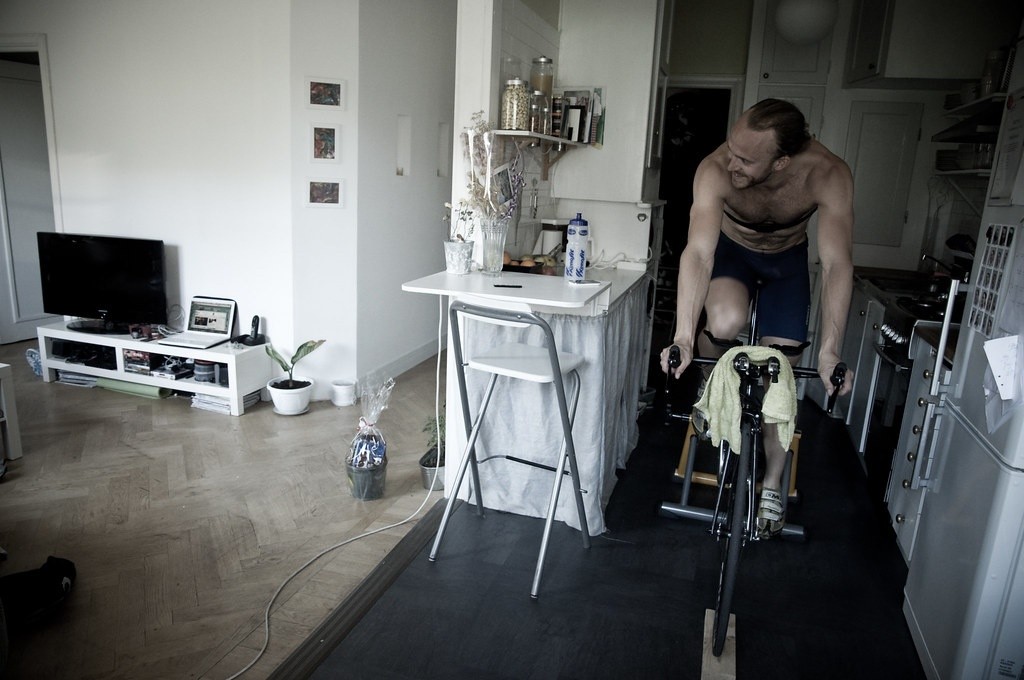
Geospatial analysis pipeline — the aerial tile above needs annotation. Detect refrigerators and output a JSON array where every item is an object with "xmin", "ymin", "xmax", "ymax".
[{"xmin": 900, "ymin": 19, "xmax": 1024, "ymax": 680}]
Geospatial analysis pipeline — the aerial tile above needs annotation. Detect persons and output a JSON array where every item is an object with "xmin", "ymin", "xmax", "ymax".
[{"xmin": 659, "ymin": 97, "xmax": 855, "ymax": 532}]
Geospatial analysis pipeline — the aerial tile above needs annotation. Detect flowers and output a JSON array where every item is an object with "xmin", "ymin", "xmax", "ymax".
[{"xmin": 442, "ymin": 199, "xmax": 480, "ymax": 242}]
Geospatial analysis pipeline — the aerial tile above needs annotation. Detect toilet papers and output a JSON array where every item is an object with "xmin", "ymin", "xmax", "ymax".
[{"xmin": 533, "ymin": 231, "xmax": 563, "ymax": 255}]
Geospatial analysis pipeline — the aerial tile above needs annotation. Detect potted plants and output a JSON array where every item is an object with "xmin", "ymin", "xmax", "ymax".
[
  {"xmin": 265, "ymin": 338, "xmax": 325, "ymax": 415},
  {"xmin": 419, "ymin": 399, "xmax": 445, "ymax": 490}
]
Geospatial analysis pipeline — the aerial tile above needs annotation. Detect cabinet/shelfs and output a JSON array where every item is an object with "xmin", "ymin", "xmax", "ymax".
[
  {"xmin": 847, "ymin": 0, "xmax": 1018, "ymax": 88},
  {"xmin": 806, "ymin": 281, "xmax": 953, "ymax": 567},
  {"xmin": 36, "ymin": 318, "xmax": 272, "ymax": 416},
  {"xmin": 929, "ymin": 93, "xmax": 1009, "ymax": 218}
]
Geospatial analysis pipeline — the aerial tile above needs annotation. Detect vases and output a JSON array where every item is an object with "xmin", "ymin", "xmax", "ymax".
[
  {"xmin": 444, "ymin": 241, "xmax": 475, "ymax": 273},
  {"xmin": 479, "ymin": 219, "xmax": 509, "ymax": 275},
  {"xmin": 346, "ymin": 454, "xmax": 387, "ymax": 501},
  {"xmin": 331, "ymin": 379, "xmax": 356, "ymax": 406}
]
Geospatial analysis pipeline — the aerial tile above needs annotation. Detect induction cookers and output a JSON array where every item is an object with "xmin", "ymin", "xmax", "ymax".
[{"xmin": 867, "ymin": 275, "xmax": 928, "ymax": 295}]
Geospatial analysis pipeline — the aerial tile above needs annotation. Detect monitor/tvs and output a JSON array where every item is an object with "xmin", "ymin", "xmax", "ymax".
[{"xmin": 36, "ymin": 231, "xmax": 168, "ymax": 335}]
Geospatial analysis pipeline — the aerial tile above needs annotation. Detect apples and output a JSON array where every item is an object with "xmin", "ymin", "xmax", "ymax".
[{"xmin": 503, "ymin": 251, "xmax": 555, "ymax": 266}]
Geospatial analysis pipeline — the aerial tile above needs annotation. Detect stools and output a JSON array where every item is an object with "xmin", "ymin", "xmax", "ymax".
[{"xmin": 427, "ymin": 294, "xmax": 591, "ymax": 600}]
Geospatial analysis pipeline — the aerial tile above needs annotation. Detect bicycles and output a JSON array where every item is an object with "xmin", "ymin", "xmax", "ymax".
[{"xmin": 662, "ymin": 272, "xmax": 848, "ymax": 657}]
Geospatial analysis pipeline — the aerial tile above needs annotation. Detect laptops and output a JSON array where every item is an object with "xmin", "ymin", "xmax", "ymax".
[{"xmin": 158, "ymin": 298, "xmax": 236, "ymax": 349}]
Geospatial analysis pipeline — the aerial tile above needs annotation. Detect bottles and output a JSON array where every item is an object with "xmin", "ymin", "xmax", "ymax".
[
  {"xmin": 501, "ymin": 55, "xmax": 563, "ymax": 137},
  {"xmin": 564, "ymin": 213, "xmax": 588, "ymax": 282},
  {"xmin": 979, "ymin": 50, "xmax": 1001, "ymax": 96},
  {"xmin": 976, "ymin": 141, "xmax": 992, "ymax": 168}
]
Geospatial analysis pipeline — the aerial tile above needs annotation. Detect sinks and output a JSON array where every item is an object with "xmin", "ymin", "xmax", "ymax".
[{"xmin": 868, "ymin": 276, "xmax": 922, "ymax": 293}]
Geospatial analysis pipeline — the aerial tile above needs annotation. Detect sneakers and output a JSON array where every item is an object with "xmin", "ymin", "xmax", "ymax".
[
  {"xmin": 691, "ymin": 375, "xmax": 712, "ymax": 441},
  {"xmin": 758, "ymin": 484, "xmax": 785, "ymax": 537}
]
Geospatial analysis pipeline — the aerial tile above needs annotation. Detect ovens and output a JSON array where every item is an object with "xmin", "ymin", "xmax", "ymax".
[{"xmin": 862, "ymin": 299, "xmax": 920, "ymax": 482}]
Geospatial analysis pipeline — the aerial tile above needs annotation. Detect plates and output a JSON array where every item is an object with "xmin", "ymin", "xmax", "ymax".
[{"xmin": 936, "ymin": 150, "xmax": 963, "ymax": 171}]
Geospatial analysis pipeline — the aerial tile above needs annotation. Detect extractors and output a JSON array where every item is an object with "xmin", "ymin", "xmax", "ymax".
[{"xmin": 931, "ymin": 108, "xmax": 1004, "ymax": 142}]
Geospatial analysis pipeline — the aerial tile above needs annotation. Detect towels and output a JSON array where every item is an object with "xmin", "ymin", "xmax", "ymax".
[{"xmin": 692, "ymin": 344, "xmax": 798, "ymax": 455}]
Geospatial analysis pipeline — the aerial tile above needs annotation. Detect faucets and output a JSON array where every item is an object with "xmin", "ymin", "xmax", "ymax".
[{"xmin": 920, "ymin": 253, "xmax": 951, "ymax": 272}]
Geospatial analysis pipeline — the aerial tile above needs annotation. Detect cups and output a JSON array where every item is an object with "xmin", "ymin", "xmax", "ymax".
[{"xmin": 332, "ymin": 379, "xmax": 357, "ymax": 406}]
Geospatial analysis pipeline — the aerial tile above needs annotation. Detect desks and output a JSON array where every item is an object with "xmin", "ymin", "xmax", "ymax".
[
  {"xmin": 401, "ymin": 262, "xmax": 649, "ymax": 538},
  {"xmin": 0, "ymin": 363, "xmax": 23, "ymax": 460}
]
